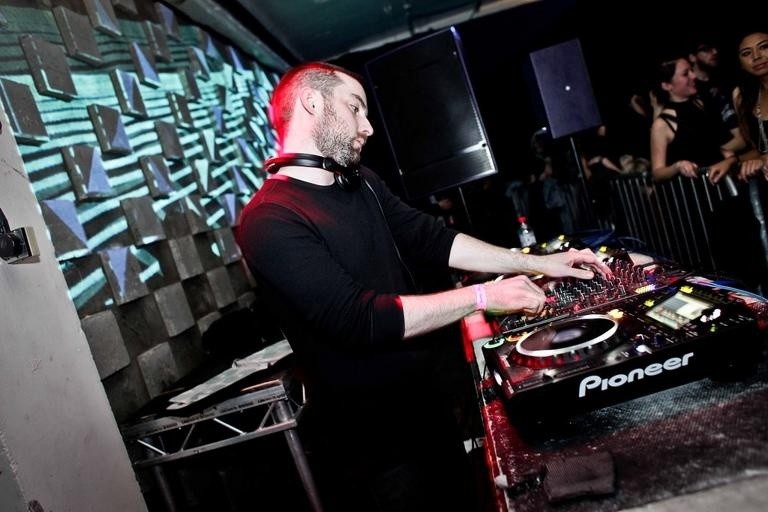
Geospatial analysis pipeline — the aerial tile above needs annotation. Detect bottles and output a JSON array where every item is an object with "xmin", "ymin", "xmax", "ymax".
[{"xmin": 516, "ymin": 215, "xmax": 536, "ymax": 247}]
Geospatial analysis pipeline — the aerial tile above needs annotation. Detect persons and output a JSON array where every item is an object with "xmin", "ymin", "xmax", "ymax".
[
  {"xmin": 427, "ymin": 29, "xmax": 767, "ymax": 298},
  {"xmin": 235, "ymin": 61, "xmax": 612, "ymax": 512}
]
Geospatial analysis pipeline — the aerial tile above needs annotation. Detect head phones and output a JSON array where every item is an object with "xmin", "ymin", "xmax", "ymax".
[{"xmin": 263, "ymin": 153, "xmax": 364, "ymax": 194}]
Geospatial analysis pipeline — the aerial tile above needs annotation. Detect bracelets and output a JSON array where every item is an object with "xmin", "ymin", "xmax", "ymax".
[{"xmin": 476, "ymin": 284, "xmax": 489, "ymax": 314}]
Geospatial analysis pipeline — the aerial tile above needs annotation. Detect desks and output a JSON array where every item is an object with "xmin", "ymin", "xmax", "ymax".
[{"xmin": 121, "ymin": 347, "xmax": 329, "ymax": 511}]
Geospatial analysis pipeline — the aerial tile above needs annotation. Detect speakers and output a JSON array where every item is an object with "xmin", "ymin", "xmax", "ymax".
[
  {"xmin": 529, "ymin": 36, "xmax": 604, "ymax": 141},
  {"xmin": 360, "ymin": 24, "xmax": 499, "ymax": 203}
]
